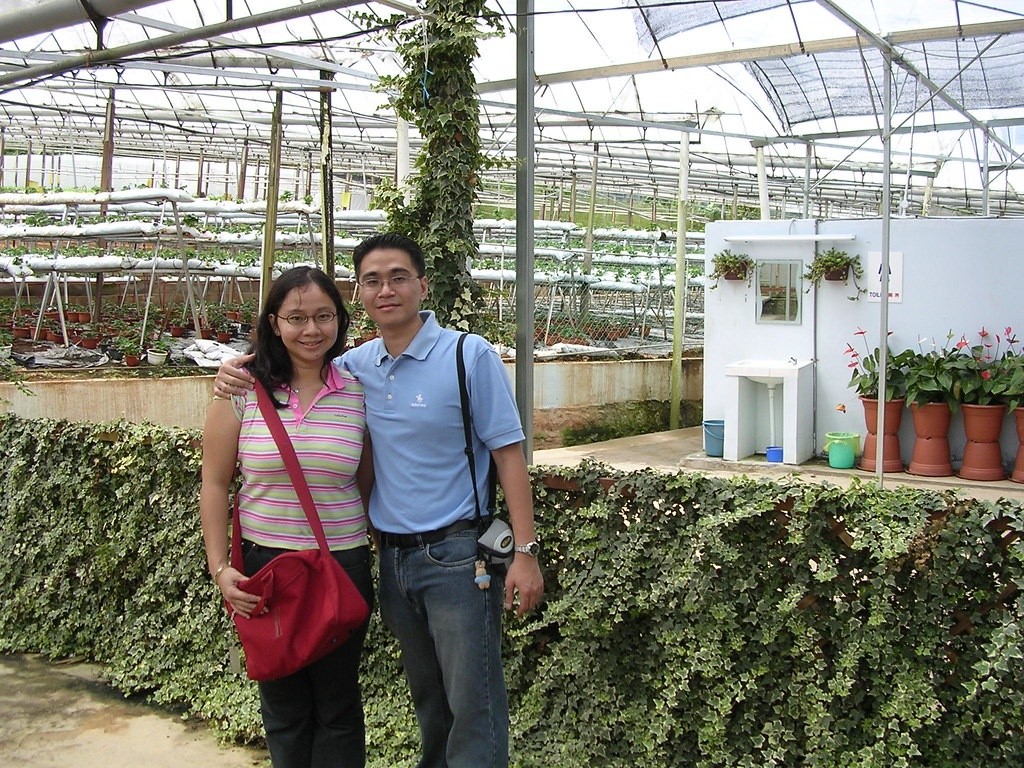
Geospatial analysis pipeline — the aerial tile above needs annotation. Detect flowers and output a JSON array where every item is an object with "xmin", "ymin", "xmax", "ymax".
[{"xmin": 843, "ymin": 326, "xmax": 1024, "ymax": 415}]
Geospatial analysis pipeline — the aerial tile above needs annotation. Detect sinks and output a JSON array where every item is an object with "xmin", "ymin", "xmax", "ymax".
[{"xmin": 739, "ymin": 361, "xmax": 784, "ymax": 385}]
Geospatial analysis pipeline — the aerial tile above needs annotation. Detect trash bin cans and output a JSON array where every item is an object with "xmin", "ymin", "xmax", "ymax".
[
  {"xmin": 826, "ymin": 432, "xmax": 861, "ymax": 469},
  {"xmin": 703, "ymin": 420, "xmax": 724, "ymax": 457}
]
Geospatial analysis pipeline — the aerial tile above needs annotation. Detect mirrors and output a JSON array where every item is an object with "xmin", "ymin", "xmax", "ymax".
[{"xmin": 754, "ymin": 258, "xmax": 801, "ymax": 325}]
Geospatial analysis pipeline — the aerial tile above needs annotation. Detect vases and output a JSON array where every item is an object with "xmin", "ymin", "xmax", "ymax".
[
  {"xmin": 904, "ymin": 401, "xmax": 956, "ymax": 476},
  {"xmin": 1008, "ymin": 406, "xmax": 1024, "ymax": 484},
  {"xmin": 955, "ymin": 403, "xmax": 1007, "ymax": 481},
  {"xmin": 856, "ymin": 395, "xmax": 905, "ymax": 472}
]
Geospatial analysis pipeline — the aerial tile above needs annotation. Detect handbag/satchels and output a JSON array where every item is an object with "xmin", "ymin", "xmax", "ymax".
[
  {"xmin": 478, "ymin": 517, "xmax": 515, "ymax": 580},
  {"xmin": 223, "ymin": 550, "xmax": 368, "ymax": 680}
]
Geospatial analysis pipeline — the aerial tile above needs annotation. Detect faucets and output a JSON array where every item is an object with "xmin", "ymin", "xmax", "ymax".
[
  {"xmin": 836, "ymin": 404, "xmax": 846, "ymax": 414},
  {"xmin": 787, "ymin": 357, "xmax": 798, "ymax": 366}
]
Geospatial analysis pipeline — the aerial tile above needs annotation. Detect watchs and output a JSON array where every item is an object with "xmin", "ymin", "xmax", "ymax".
[{"xmin": 514, "ymin": 541, "xmax": 540, "ymax": 556}]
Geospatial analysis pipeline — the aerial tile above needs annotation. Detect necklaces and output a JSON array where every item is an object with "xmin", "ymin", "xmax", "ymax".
[{"xmin": 292, "ymin": 380, "xmax": 322, "ymax": 393}]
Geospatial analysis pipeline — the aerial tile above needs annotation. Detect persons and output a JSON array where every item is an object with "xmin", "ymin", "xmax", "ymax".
[
  {"xmin": 215, "ymin": 234, "xmax": 544, "ymax": 768},
  {"xmin": 200, "ymin": 265, "xmax": 410, "ymax": 768}
]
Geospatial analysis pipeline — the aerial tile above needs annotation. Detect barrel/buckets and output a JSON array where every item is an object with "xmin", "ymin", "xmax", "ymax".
[
  {"xmin": 702, "ymin": 420, "xmax": 724, "ymax": 456},
  {"xmin": 766, "ymin": 446, "xmax": 783, "ymax": 461}
]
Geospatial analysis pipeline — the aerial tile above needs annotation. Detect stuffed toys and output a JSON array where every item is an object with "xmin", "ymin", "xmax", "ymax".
[{"xmin": 475, "ymin": 561, "xmax": 491, "ymax": 589}]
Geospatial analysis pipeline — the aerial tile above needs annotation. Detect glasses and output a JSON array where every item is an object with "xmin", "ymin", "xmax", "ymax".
[
  {"xmin": 357, "ymin": 276, "xmax": 418, "ymax": 290},
  {"xmin": 278, "ymin": 313, "xmax": 338, "ymax": 325}
]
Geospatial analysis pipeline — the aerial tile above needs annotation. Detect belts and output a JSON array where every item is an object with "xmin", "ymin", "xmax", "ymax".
[{"xmin": 370, "ymin": 519, "xmax": 475, "ymax": 548}]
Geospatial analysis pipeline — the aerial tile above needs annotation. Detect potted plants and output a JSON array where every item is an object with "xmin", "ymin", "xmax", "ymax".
[
  {"xmin": 709, "ymin": 249, "xmax": 765, "ymax": 303},
  {"xmin": 342, "ymin": 299, "xmax": 378, "ymax": 349},
  {"xmin": 0, "ymin": 296, "xmax": 258, "ymax": 368},
  {"xmin": 803, "ymin": 247, "xmax": 867, "ymax": 300},
  {"xmin": 499, "ymin": 315, "xmax": 653, "ymax": 349}
]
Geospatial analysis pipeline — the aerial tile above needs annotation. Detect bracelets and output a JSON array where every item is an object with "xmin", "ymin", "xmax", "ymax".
[{"xmin": 215, "ymin": 564, "xmax": 229, "ymax": 585}]
